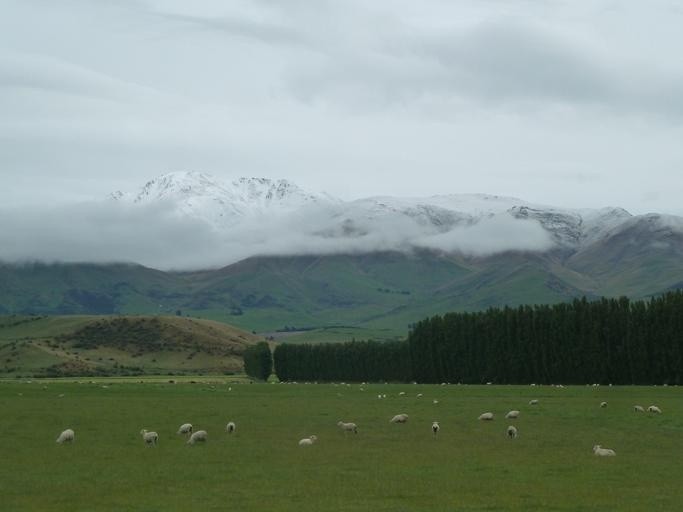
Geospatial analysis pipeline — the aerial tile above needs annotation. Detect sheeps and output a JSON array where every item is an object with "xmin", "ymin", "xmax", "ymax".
[
  {"xmin": 337, "ymin": 421, "xmax": 361, "ymax": 441},
  {"xmin": 505, "ymin": 410, "xmax": 520, "ymax": 440},
  {"xmin": 390, "ymin": 414, "xmax": 409, "ymax": 424},
  {"xmin": 56, "ymin": 429, "xmax": 74, "ymax": 445},
  {"xmin": 600, "ymin": 402, "xmax": 607, "ymax": 407},
  {"xmin": 529, "ymin": 400, "xmax": 539, "ymax": 405},
  {"xmin": 375, "ymin": 391, "xmax": 439, "ymax": 404},
  {"xmin": 140, "ymin": 422, "xmax": 236, "ymax": 449},
  {"xmin": 634, "ymin": 406, "xmax": 661, "ymax": 414},
  {"xmin": 478, "ymin": 412, "xmax": 493, "ymax": 420},
  {"xmin": 299, "ymin": 435, "xmax": 317, "ymax": 446},
  {"xmin": 593, "ymin": 445, "xmax": 616, "ymax": 456},
  {"xmin": 432, "ymin": 422, "xmax": 440, "ymax": 439}
]
